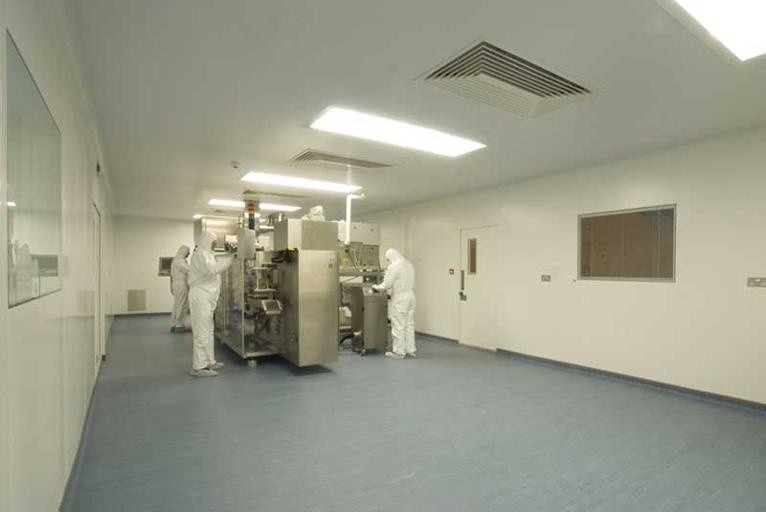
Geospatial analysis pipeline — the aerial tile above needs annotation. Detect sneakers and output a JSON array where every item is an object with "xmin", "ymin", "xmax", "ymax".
[
  {"xmin": 386, "ymin": 351, "xmax": 403, "ymax": 358},
  {"xmin": 190, "ymin": 368, "xmax": 216, "ymax": 377},
  {"xmin": 407, "ymin": 354, "xmax": 415, "ymax": 357},
  {"xmin": 209, "ymin": 362, "xmax": 223, "ymax": 370}
]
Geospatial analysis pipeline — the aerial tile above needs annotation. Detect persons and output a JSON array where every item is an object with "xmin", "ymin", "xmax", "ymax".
[
  {"xmin": 187, "ymin": 231, "xmax": 231, "ymax": 377},
  {"xmin": 170, "ymin": 246, "xmax": 190, "ymax": 332},
  {"xmin": 372, "ymin": 248, "xmax": 416, "ymax": 359}
]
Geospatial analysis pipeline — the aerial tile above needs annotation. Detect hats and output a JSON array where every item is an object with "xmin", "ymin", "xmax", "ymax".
[{"xmin": 170, "ymin": 326, "xmax": 192, "ymax": 333}]
